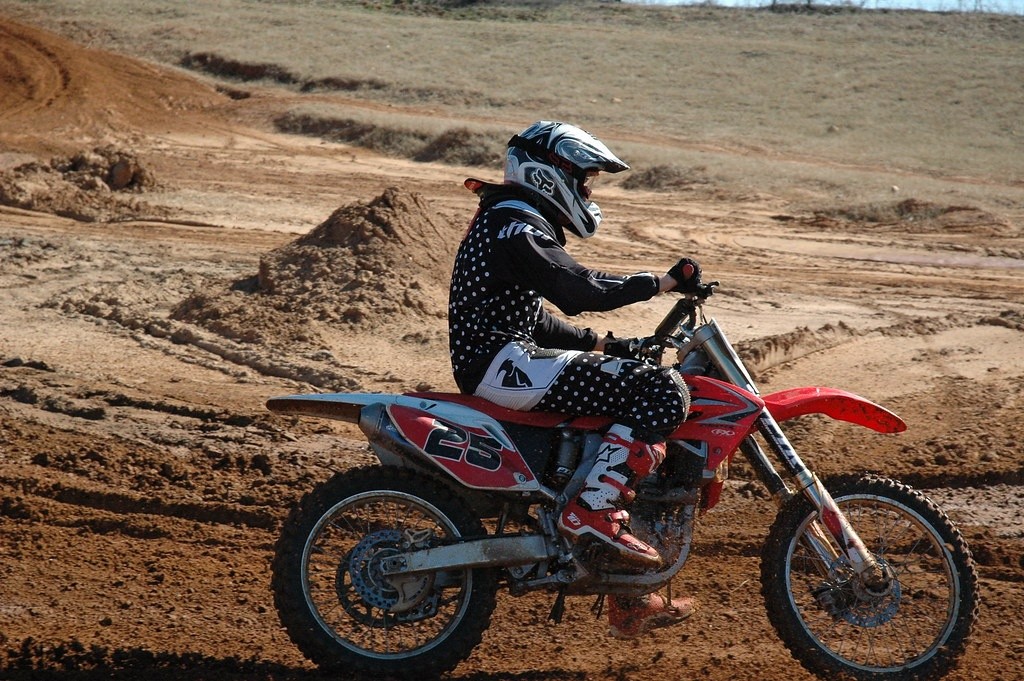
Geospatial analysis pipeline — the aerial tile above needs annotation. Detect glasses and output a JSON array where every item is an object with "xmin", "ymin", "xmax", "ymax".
[{"xmin": 576, "ymin": 167, "xmax": 599, "ymax": 198}]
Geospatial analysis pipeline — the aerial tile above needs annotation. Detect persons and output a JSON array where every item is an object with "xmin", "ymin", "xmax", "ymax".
[{"xmin": 448, "ymin": 119, "xmax": 702, "ymax": 638}]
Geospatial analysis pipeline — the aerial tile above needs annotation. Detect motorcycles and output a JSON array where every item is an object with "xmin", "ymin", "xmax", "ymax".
[{"xmin": 264, "ymin": 265, "xmax": 979, "ymax": 681}]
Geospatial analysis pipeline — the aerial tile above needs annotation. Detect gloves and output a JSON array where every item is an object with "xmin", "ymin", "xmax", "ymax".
[
  {"xmin": 666, "ymin": 257, "xmax": 703, "ymax": 293},
  {"xmin": 603, "ymin": 331, "xmax": 639, "ymax": 358}
]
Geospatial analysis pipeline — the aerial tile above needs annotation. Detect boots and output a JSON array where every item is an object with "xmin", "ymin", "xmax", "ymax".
[
  {"xmin": 606, "ymin": 590, "xmax": 701, "ymax": 639},
  {"xmin": 556, "ymin": 422, "xmax": 666, "ymax": 576}
]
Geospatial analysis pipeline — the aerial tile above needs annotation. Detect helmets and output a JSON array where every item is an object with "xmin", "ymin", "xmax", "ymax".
[{"xmin": 503, "ymin": 120, "xmax": 631, "ymax": 239}]
